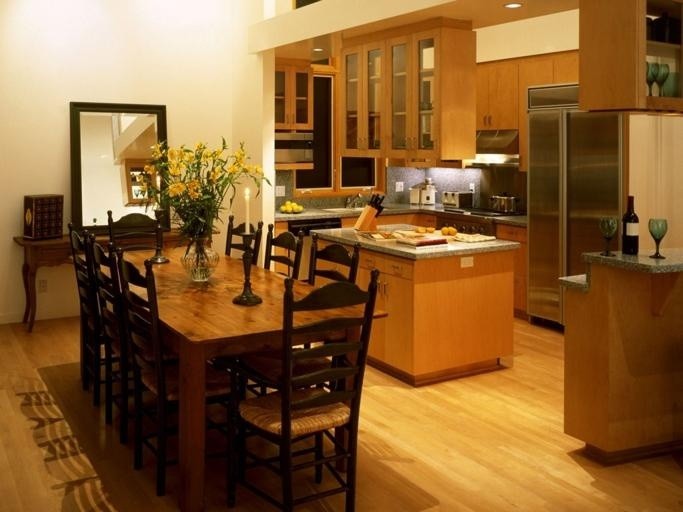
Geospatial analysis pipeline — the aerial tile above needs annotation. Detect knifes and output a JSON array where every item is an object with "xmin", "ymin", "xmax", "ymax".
[{"xmin": 368, "ymin": 193, "xmax": 385, "ymax": 217}]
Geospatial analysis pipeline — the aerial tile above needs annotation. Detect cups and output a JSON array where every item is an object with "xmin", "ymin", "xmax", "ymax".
[{"xmin": 663, "ymin": 72, "xmax": 679, "ymax": 97}]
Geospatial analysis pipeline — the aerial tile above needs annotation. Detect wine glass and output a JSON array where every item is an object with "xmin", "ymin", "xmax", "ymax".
[
  {"xmin": 599, "ymin": 216, "xmax": 618, "ymax": 256},
  {"xmin": 651, "ymin": 63, "xmax": 669, "ymax": 95},
  {"xmin": 648, "ymin": 219, "xmax": 668, "ymax": 259},
  {"xmin": 647, "ymin": 62, "xmax": 658, "ymax": 97}
]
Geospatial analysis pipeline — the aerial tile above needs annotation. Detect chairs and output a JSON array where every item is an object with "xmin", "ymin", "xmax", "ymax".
[
  {"xmin": 264, "ymin": 224, "xmax": 305, "ymax": 282},
  {"xmin": 68, "ymin": 223, "xmax": 143, "ymax": 407},
  {"xmin": 229, "ymin": 270, "xmax": 380, "ymax": 511},
  {"xmin": 238, "ymin": 235, "xmax": 363, "ymax": 452},
  {"xmin": 91, "ymin": 234, "xmax": 179, "ymax": 443},
  {"xmin": 116, "ymin": 247, "xmax": 241, "ymax": 496},
  {"xmin": 225, "ymin": 215, "xmax": 264, "ymax": 266}
]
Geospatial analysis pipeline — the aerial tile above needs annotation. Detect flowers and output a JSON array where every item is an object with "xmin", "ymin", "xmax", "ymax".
[{"xmin": 132, "ymin": 136, "xmax": 272, "ymax": 265}]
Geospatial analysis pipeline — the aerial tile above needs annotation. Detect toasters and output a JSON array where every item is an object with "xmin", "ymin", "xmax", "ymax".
[{"xmin": 441, "ymin": 191, "xmax": 472, "ymax": 209}]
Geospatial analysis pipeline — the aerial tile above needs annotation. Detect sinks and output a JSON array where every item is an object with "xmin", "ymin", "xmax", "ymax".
[{"xmin": 322, "ymin": 206, "xmax": 365, "ymax": 212}]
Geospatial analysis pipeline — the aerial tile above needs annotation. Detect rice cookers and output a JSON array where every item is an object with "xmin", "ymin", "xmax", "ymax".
[{"xmin": 409, "ymin": 178, "xmax": 436, "ymax": 204}]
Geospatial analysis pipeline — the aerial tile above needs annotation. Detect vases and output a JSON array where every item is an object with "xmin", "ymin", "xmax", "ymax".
[{"xmin": 181, "ymin": 235, "xmax": 219, "ymax": 282}]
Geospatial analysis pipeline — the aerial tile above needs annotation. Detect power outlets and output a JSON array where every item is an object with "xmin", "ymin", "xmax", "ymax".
[
  {"xmin": 471, "ymin": 183, "xmax": 476, "ymax": 194},
  {"xmin": 395, "ymin": 182, "xmax": 404, "ymax": 192},
  {"xmin": 276, "ymin": 186, "xmax": 286, "ymax": 198}
]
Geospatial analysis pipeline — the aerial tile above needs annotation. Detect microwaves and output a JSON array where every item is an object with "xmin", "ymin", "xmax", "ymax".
[{"xmin": 274, "ymin": 133, "xmax": 314, "ymax": 163}]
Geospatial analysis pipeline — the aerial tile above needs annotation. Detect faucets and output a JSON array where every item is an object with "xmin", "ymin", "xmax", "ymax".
[{"xmin": 344, "ymin": 193, "xmax": 362, "ymax": 208}]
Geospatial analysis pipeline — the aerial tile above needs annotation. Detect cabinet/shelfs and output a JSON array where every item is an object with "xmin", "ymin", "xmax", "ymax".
[
  {"xmin": 311, "ymin": 224, "xmax": 520, "ymax": 384},
  {"xmin": 519, "ymin": 56, "xmax": 580, "ymax": 172},
  {"xmin": 341, "ymin": 36, "xmax": 386, "ymax": 159},
  {"xmin": 560, "ymin": 250, "xmax": 682, "ymax": 465},
  {"xmin": 495, "ymin": 222, "xmax": 527, "ymax": 242},
  {"xmin": 13, "ymin": 230, "xmax": 220, "ymax": 334},
  {"xmin": 495, "ymin": 238, "xmax": 528, "ymax": 323},
  {"xmin": 386, "ymin": 27, "xmax": 476, "ymax": 161},
  {"xmin": 578, "ymin": 1, "xmax": 683, "ymax": 112},
  {"xmin": 275, "ymin": 65, "xmax": 314, "ymax": 131},
  {"xmin": 477, "ymin": 63, "xmax": 519, "ymax": 131}
]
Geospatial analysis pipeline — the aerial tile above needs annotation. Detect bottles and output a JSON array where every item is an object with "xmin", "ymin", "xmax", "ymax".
[{"xmin": 619, "ymin": 196, "xmax": 639, "ymax": 255}]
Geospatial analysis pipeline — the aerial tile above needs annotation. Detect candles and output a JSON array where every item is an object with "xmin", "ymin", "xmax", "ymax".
[{"xmin": 245, "ymin": 187, "xmax": 251, "ymax": 235}]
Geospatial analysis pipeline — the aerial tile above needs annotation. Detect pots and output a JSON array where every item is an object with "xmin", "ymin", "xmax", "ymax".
[{"xmin": 487, "ymin": 192, "xmax": 521, "ymax": 212}]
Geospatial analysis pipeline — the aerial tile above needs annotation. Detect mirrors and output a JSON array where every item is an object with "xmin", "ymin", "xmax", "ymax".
[{"xmin": 70, "ymin": 102, "xmax": 170, "ymax": 234}]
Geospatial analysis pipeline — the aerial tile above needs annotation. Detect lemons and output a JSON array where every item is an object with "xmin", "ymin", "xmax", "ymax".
[
  {"xmin": 297, "ymin": 206, "xmax": 303, "ymax": 212},
  {"xmin": 292, "ymin": 206, "xmax": 297, "ymax": 212},
  {"xmin": 280, "ymin": 206, "xmax": 286, "ymax": 213},
  {"xmin": 286, "ymin": 201, "xmax": 291, "ymax": 206},
  {"xmin": 291, "ymin": 203, "xmax": 297, "ymax": 205},
  {"xmin": 286, "ymin": 206, "xmax": 292, "ymax": 212}
]
